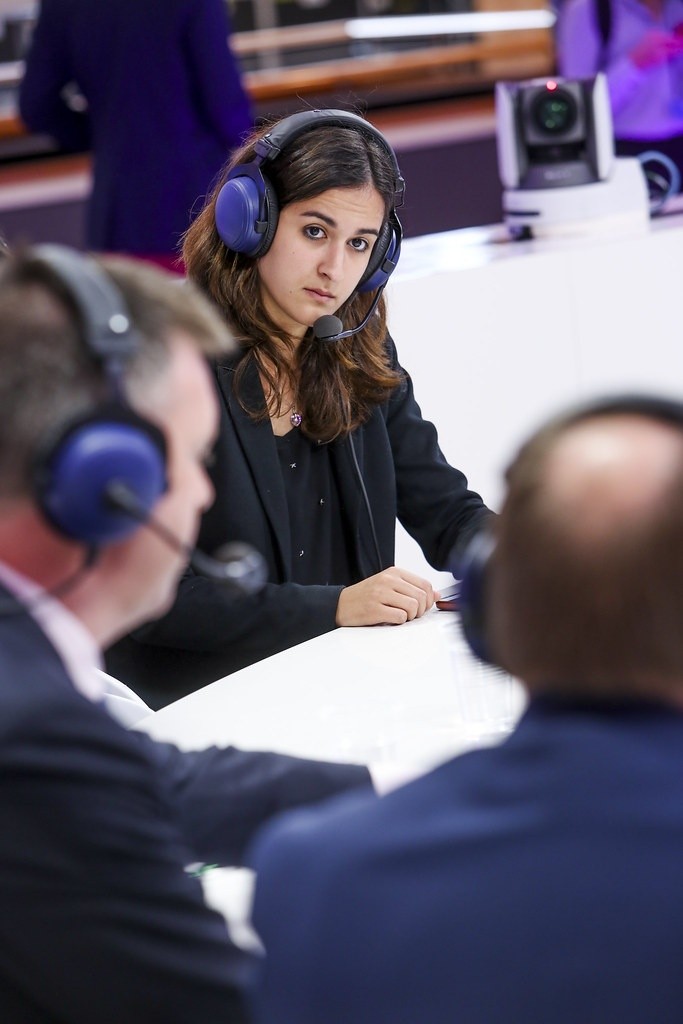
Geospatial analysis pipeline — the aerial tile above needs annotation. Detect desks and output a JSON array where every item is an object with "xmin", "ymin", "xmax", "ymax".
[
  {"xmin": 156, "ymin": 582, "xmax": 530, "ymax": 957},
  {"xmin": 382, "ymin": 194, "xmax": 682, "ymax": 590}
]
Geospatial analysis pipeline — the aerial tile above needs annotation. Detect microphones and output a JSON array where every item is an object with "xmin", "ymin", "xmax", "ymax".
[
  {"xmin": 312, "ymin": 281, "xmax": 388, "ymax": 344},
  {"xmin": 107, "ymin": 482, "xmax": 266, "ymax": 595}
]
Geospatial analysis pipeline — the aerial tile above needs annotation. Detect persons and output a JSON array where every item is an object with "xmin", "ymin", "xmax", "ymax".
[
  {"xmin": 21, "ymin": 0, "xmax": 253, "ymax": 276},
  {"xmin": 250, "ymin": 397, "xmax": 683, "ymax": 1024},
  {"xmin": 0, "ymin": 244, "xmax": 382, "ymax": 1024},
  {"xmin": 557, "ymin": 0, "xmax": 683, "ymax": 198},
  {"xmin": 101, "ymin": 109, "xmax": 499, "ymax": 712}
]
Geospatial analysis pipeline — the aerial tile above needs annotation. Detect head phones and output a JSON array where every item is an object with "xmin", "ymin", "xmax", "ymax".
[
  {"xmin": 0, "ymin": 242, "xmax": 171, "ymax": 555},
  {"xmin": 216, "ymin": 107, "xmax": 403, "ymax": 293},
  {"xmin": 453, "ymin": 393, "xmax": 682, "ymax": 670}
]
